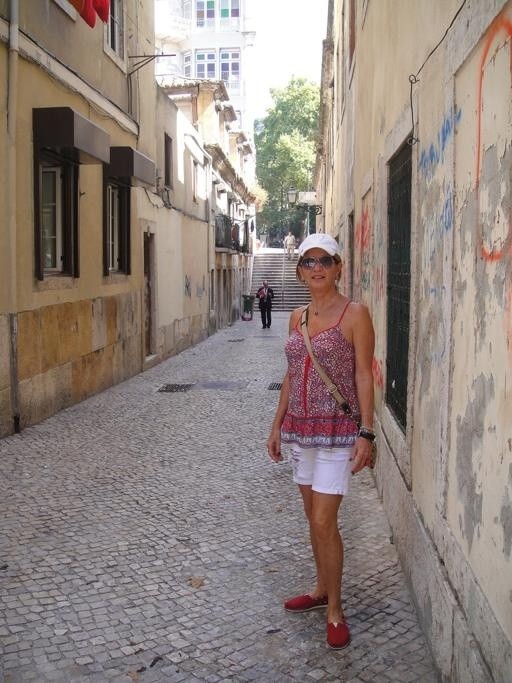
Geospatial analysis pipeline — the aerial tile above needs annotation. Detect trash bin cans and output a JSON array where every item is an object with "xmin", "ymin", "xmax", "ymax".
[{"xmin": 242, "ymin": 294, "xmax": 255, "ymax": 321}]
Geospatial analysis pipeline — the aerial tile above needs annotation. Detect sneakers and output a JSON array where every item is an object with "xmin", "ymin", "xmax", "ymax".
[
  {"xmin": 326, "ymin": 612, "xmax": 349, "ymax": 650},
  {"xmin": 284, "ymin": 593, "xmax": 328, "ymax": 613}
]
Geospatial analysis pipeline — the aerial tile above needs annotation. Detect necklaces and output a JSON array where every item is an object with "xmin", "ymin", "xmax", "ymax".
[{"xmin": 315, "ymin": 312, "xmax": 318, "ymax": 315}]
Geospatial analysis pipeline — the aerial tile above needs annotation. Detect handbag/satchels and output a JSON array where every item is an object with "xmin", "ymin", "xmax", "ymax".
[{"xmin": 369, "ymin": 438, "xmax": 376, "ymax": 468}]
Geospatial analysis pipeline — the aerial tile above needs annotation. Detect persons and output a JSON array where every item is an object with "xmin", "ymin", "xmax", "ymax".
[
  {"xmin": 266, "ymin": 233, "xmax": 377, "ymax": 650},
  {"xmin": 284, "ymin": 231, "xmax": 296, "ymax": 261},
  {"xmin": 256, "ymin": 280, "xmax": 274, "ymax": 329}
]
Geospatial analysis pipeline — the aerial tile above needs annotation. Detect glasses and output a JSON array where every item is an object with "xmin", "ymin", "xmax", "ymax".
[{"xmin": 299, "ymin": 256, "xmax": 333, "ymax": 269}]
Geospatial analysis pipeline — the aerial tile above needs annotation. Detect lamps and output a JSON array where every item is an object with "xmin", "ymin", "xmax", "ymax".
[{"xmin": 287, "ymin": 185, "xmax": 322, "ymax": 215}]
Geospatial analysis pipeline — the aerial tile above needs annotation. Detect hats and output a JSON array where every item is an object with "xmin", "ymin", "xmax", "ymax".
[{"xmin": 297, "ymin": 232, "xmax": 339, "ymax": 256}]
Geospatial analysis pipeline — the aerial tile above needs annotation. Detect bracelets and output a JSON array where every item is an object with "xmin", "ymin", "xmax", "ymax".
[{"xmin": 358, "ymin": 426, "xmax": 375, "ymax": 441}]
